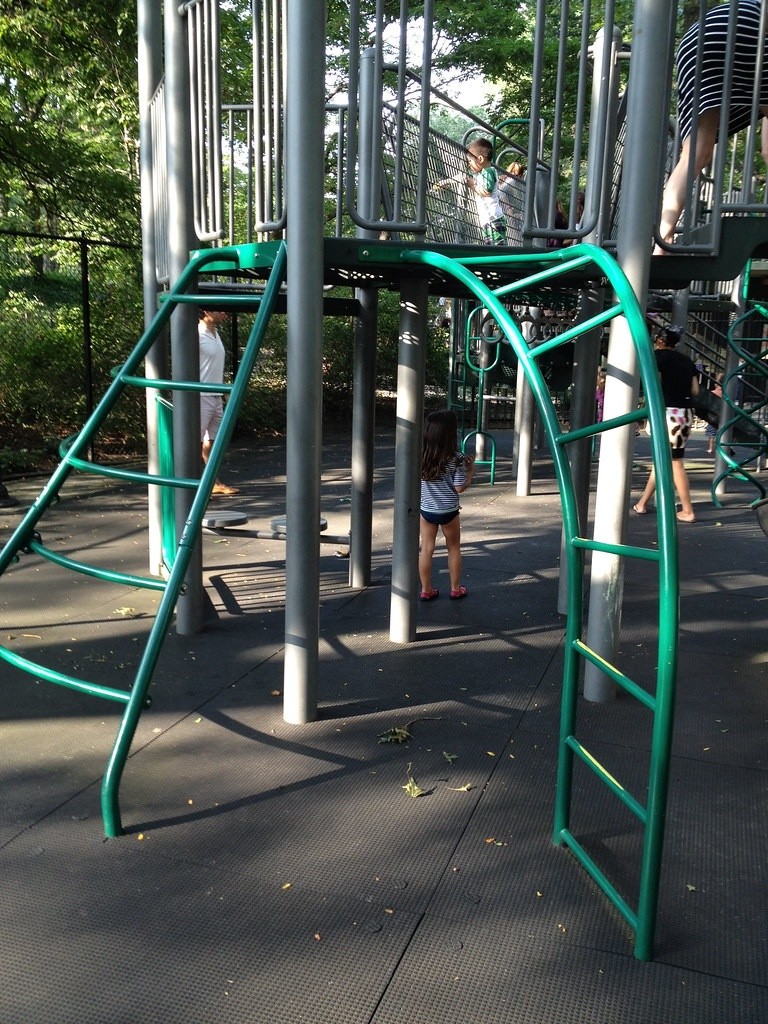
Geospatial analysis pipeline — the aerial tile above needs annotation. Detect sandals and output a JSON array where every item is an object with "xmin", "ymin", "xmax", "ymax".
[
  {"xmin": 420, "ymin": 588, "xmax": 439, "ymax": 601},
  {"xmin": 450, "ymin": 585, "xmax": 467, "ymax": 599}
]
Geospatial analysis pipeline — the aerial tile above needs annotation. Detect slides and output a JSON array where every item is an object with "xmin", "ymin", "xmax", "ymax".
[{"xmin": 600, "ymin": 334, "xmax": 768, "ymax": 451}]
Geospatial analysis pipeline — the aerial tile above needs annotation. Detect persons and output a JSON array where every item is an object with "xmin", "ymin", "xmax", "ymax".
[
  {"xmin": 430, "ymin": 138, "xmax": 507, "ymax": 245},
  {"xmin": 418, "ymin": 410, "xmax": 476, "ymax": 602},
  {"xmin": 706, "ymin": 374, "xmax": 736, "ymax": 456},
  {"xmin": 633, "ymin": 326, "xmax": 696, "ymax": 522},
  {"xmin": 499, "ymin": 161, "xmax": 585, "ymax": 248},
  {"xmin": 649, "ymin": 0, "xmax": 768, "ymax": 255},
  {"xmin": 198, "ymin": 310, "xmax": 240, "ymax": 494}
]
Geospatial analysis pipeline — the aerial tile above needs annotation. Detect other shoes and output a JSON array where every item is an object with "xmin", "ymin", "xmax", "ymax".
[
  {"xmin": 676, "ymin": 512, "xmax": 695, "ymax": 522},
  {"xmin": 633, "ymin": 505, "xmax": 646, "ymax": 513}
]
